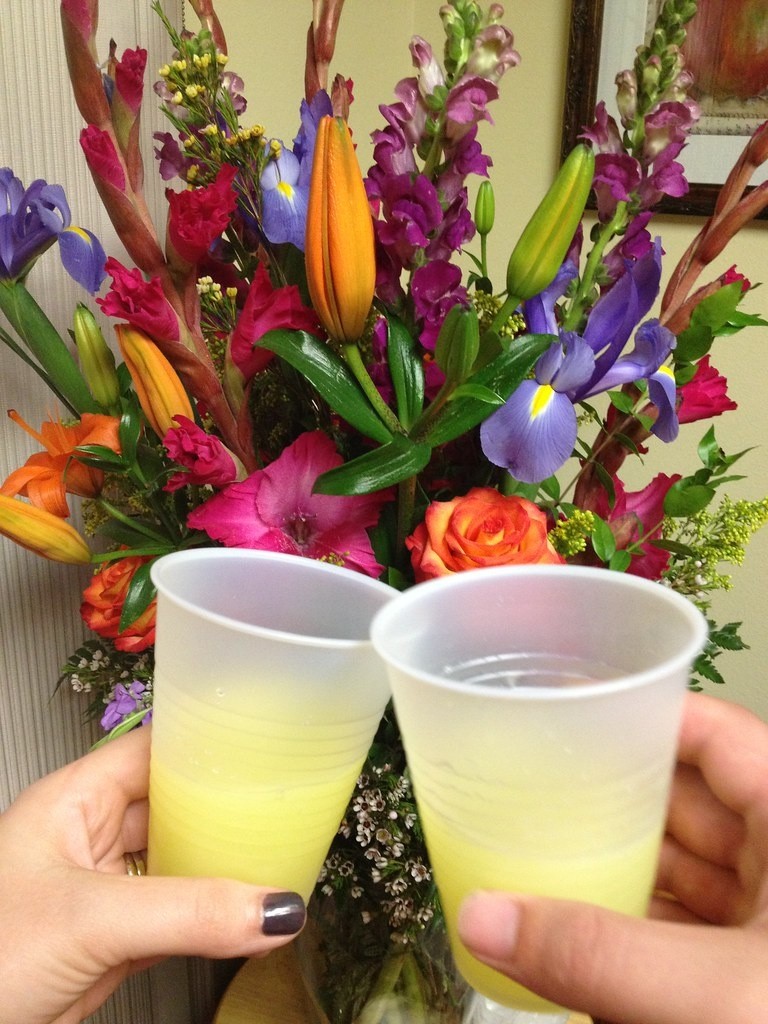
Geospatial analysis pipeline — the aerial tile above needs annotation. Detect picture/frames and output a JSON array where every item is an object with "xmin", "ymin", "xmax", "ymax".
[{"xmin": 561, "ymin": 0, "xmax": 768, "ymax": 221}]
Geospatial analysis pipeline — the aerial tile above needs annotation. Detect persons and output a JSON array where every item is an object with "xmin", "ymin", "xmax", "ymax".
[
  {"xmin": 457, "ymin": 690, "xmax": 768, "ymax": 1024},
  {"xmin": 1, "ymin": 726, "xmax": 309, "ymax": 1024}
]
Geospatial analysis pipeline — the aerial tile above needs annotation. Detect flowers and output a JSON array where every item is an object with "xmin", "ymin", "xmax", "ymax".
[{"xmin": 0, "ymin": 0, "xmax": 768, "ymax": 1023}]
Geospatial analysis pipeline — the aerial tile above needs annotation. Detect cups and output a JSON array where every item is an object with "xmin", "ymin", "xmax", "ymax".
[
  {"xmin": 145, "ymin": 547, "xmax": 401, "ymax": 910},
  {"xmin": 369, "ymin": 564, "xmax": 708, "ymax": 1013}
]
